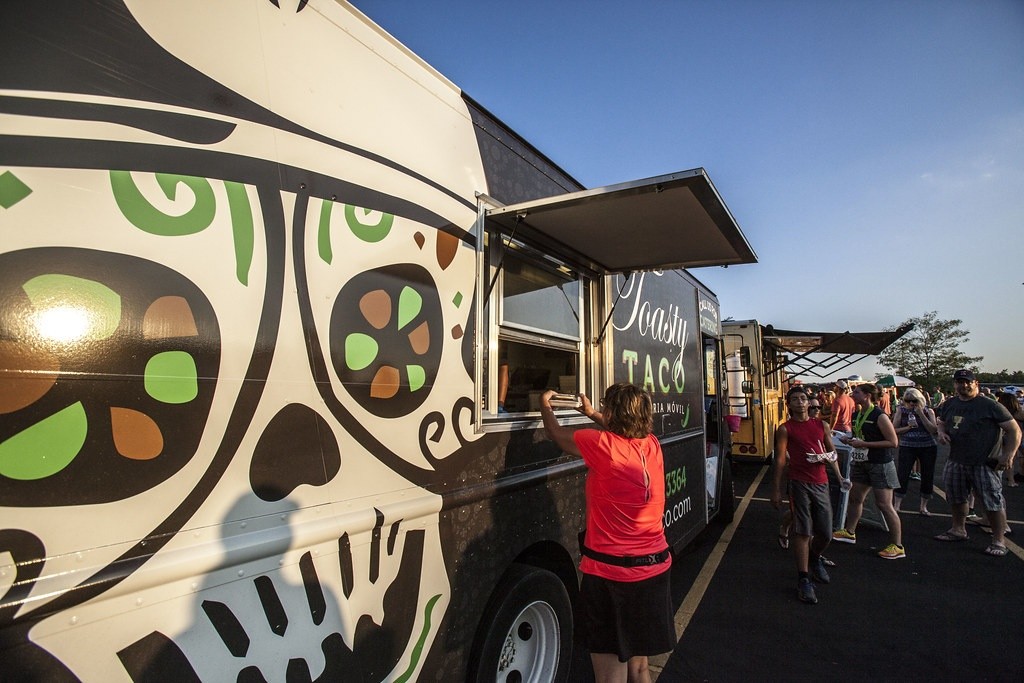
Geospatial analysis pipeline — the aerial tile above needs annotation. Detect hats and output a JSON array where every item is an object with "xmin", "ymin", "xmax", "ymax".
[
  {"xmin": 836, "ymin": 380, "xmax": 847, "ymax": 389},
  {"xmin": 953, "ymin": 369, "xmax": 976, "ymax": 381}
]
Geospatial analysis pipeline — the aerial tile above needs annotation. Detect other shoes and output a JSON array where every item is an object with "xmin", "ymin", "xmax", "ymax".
[
  {"xmin": 920, "ymin": 508, "xmax": 930, "ymax": 516},
  {"xmin": 908, "ymin": 471, "xmax": 921, "ymax": 480}
]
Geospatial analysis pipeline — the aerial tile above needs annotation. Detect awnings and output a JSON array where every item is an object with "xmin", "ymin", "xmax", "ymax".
[
  {"xmin": 763, "ymin": 324, "xmax": 914, "ymax": 383},
  {"xmin": 486, "ymin": 167, "xmax": 758, "ymax": 344}
]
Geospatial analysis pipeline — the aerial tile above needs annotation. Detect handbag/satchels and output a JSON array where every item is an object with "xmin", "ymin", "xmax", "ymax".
[{"xmin": 578, "ymin": 528, "xmax": 669, "ymax": 567}]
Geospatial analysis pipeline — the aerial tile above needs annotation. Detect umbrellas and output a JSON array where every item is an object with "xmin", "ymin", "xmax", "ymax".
[{"xmin": 875, "ymin": 376, "xmax": 916, "ymax": 399}]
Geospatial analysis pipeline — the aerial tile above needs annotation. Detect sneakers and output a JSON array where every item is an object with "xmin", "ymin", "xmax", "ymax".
[
  {"xmin": 795, "ymin": 577, "xmax": 818, "ymax": 604},
  {"xmin": 831, "ymin": 529, "xmax": 856, "ymax": 544},
  {"xmin": 808, "ymin": 556, "xmax": 829, "ymax": 584},
  {"xmin": 877, "ymin": 544, "xmax": 906, "ymax": 560}
]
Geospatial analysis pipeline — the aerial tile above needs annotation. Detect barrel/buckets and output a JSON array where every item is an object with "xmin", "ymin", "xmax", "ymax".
[{"xmin": 724, "ymin": 415, "xmax": 741, "ymax": 432}]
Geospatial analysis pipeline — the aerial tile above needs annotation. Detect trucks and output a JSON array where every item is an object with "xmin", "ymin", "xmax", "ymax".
[
  {"xmin": 723, "ymin": 318, "xmax": 917, "ymax": 469},
  {"xmin": 0, "ymin": 0, "xmax": 760, "ymax": 683}
]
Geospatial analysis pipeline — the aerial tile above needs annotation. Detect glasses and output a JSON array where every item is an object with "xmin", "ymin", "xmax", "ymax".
[
  {"xmin": 810, "ymin": 405, "xmax": 821, "ymax": 409},
  {"xmin": 904, "ymin": 399, "xmax": 918, "ymax": 404},
  {"xmin": 599, "ymin": 397, "xmax": 609, "ymax": 408}
]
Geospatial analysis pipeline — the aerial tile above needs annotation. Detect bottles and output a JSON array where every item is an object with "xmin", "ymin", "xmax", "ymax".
[
  {"xmin": 908, "ymin": 412, "xmax": 918, "ymax": 428},
  {"xmin": 840, "ymin": 479, "xmax": 852, "ymax": 493}
]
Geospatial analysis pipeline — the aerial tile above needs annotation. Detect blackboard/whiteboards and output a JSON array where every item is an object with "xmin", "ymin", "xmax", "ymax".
[{"xmin": 825, "ymin": 447, "xmax": 850, "ymax": 532}]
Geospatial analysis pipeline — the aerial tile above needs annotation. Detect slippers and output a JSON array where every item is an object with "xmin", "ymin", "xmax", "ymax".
[
  {"xmin": 820, "ymin": 556, "xmax": 836, "ymax": 568},
  {"xmin": 778, "ymin": 525, "xmax": 790, "ymax": 550},
  {"xmin": 984, "ymin": 543, "xmax": 1009, "ymax": 556},
  {"xmin": 934, "ymin": 531, "xmax": 969, "ymax": 542}
]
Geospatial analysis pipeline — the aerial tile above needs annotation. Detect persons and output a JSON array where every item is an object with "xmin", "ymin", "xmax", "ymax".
[
  {"xmin": 829, "ymin": 380, "xmax": 851, "ymax": 432},
  {"xmin": 938, "ymin": 370, "xmax": 1022, "ymax": 556},
  {"xmin": 893, "ymin": 388, "xmax": 938, "ymax": 515},
  {"xmin": 998, "ymin": 393, "xmax": 1024, "ymax": 487},
  {"xmin": 876, "ymin": 384, "xmax": 890, "ymax": 416},
  {"xmin": 933, "ymin": 386, "xmax": 944, "ymax": 408},
  {"xmin": 770, "ymin": 388, "xmax": 852, "ymax": 603},
  {"xmin": 498, "ymin": 352, "xmax": 508, "ymax": 413},
  {"xmin": 980, "ymin": 383, "xmax": 1024, "ymax": 405},
  {"xmin": 832, "ymin": 384, "xmax": 906, "ymax": 559},
  {"xmin": 805, "ymin": 385, "xmax": 954, "ymax": 424},
  {"xmin": 778, "ymin": 396, "xmax": 836, "ymax": 566},
  {"xmin": 916, "ymin": 384, "xmax": 931, "ymax": 408},
  {"xmin": 540, "ymin": 382, "xmax": 677, "ymax": 682}
]
